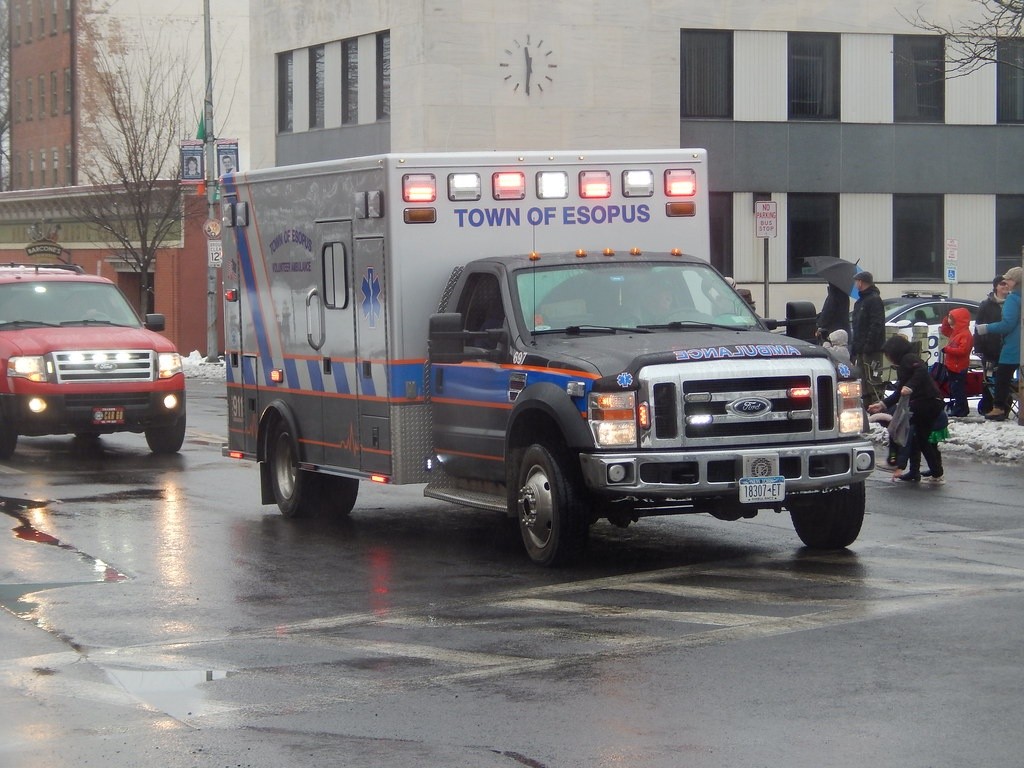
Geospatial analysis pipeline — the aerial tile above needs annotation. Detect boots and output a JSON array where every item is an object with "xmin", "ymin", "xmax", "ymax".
[
  {"xmin": 920, "ymin": 456, "xmax": 943, "ymax": 478},
  {"xmin": 985, "ymin": 399, "xmax": 1010, "ymax": 419},
  {"xmin": 901, "ymin": 463, "xmax": 921, "ymax": 481}
]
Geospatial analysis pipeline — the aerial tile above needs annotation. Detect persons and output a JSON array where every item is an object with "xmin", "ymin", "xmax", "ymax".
[
  {"xmin": 626, "ymin": 275, "xmax": 677, "ymax": 327},
  {"xmin": 711, "ymin": 277, "xmax": 756, "ymax": 320},
  {"xmin": 941, "ymin": 308, "xmax": 974, "ymax": 418},
  {"xmin": 974, "ymin": 265, "xmax": 1022, "ymax": 420},
  {"xmin": 184, "ymin": 157, "xmax": 200, "ymax": 176},
  {"xmin": 220, "ymin": 155, "xmax": 236, "ymax": 176},
  {"xmin": 813, "ymin": 270, "xmax": 949, "ymax": 483}
]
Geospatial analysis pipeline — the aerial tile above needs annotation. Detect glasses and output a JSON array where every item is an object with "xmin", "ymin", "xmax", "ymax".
[{"xmin": 998, "ymin": 282, "xmax": 1007, "ymax": 286}]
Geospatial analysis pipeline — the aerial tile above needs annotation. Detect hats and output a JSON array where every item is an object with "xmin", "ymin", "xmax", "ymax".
[
  {"xmin": 852, "ymin": 270, "xmax": 873, "ymax": 283},
  {"xmin": 1002, "ymin": 267, "xmax": 1024, "ymax": 284},
  {"xmin": 736, "ymin": 289, "xmax": 752, "ymax": 306},
  {"xmin": 993, "ymin": 276, "xmax": 1005, "ymax": 287},
  {"xmin": 725, "ymin": 277, "xmax": 738, "ymax": 289}
]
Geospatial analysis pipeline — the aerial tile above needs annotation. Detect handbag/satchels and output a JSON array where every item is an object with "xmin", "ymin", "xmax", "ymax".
[
  {"xmin": 929, "ymin": 349, "xmax": 952, "ymax": 398},
  {"xmin": 887, "ymin": 395, "xmax": 911, "ymax": 448}
]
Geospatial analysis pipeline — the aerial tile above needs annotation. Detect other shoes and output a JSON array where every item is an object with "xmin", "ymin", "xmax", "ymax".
[
  {"xmin": 876, "ymin": 457, "xmax": 899, "ymax": 473},
  {"xmin": 948, "ymin": 412, "xmax": 969, "ymax": 417}
]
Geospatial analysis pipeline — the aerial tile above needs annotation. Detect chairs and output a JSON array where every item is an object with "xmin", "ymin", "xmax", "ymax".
[
  {"xmin": 865, "ymin": 363, "xmax": 897, "ymax": 404},
  {"xmin": 982, "ymin": 354, "xmax": 1019, "ymax": 421},
  {"xmin": 914, "ymin": 307, "xmax": 939, "ymax": 324},
  {"xmin": 475, "ymin": 299, "xmax": 505, "ymax": 351}
]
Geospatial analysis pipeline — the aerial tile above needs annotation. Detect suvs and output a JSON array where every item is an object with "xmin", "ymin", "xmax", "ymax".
[{"xmin": 0, "ymin": 263, "xmax": 188, "ymax": 461}]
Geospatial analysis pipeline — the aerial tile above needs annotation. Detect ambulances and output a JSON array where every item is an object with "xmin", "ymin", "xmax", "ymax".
[{"xmin": 216, "ymin": 149, "xmax": 876, "ymax": 565}]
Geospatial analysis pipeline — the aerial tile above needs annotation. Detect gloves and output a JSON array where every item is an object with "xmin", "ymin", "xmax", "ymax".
[{"xmin": 976, "ymin": 324, "xmax": 988, "ymax": 335}]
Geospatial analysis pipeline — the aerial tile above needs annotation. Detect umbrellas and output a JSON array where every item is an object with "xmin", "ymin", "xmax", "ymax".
[{"xmin": 808, "ymin": 254, "xmax": 866, "ymax": 300}]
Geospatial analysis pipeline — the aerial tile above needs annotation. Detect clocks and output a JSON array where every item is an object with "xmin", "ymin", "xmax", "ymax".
[{"xmin": 499, "ymin": 35, "xmax": 557, "ymax": 96}]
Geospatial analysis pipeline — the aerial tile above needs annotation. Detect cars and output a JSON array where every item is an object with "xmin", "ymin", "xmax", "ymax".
[{"xmin": 884, "ymin": 291, "xmax": 980, "ymax": 327}]
